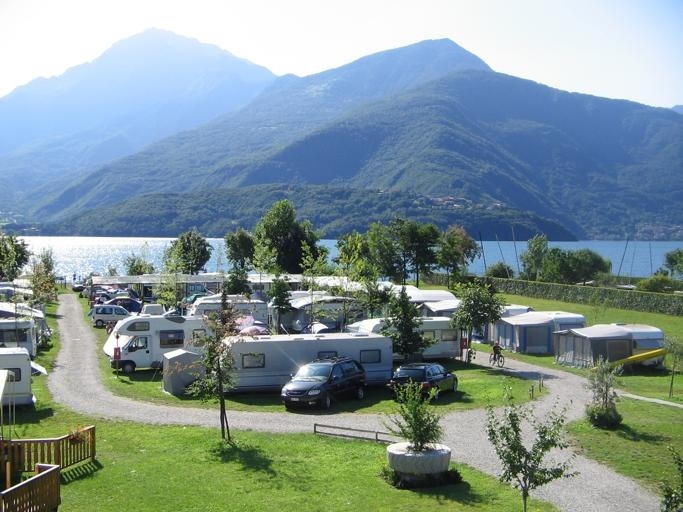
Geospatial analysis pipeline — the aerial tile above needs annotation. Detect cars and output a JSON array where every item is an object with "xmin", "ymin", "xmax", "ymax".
[{"xmin": 392, "ymin": 363, "xmax": 457, "ymax": 401}]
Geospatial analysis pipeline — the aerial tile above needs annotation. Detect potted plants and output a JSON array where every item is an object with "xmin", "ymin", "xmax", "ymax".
[{"xmin": 378, "ymin": 378, "xmax": 453, "ymax": 489}]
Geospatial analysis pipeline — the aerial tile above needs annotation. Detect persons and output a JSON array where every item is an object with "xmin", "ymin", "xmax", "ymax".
[{"xmin": 493, "ymin": 341, "xmax": 505, "ymax": 360}]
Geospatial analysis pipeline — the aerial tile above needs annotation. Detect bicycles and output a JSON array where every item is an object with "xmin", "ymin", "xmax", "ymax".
[{"xmin": 490, "ymin": 349, "xmax": 504, "ymax": 368}]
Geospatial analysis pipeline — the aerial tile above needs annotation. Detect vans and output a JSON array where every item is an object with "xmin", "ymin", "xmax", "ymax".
[
  {"xmin": 0, "ymin": 316, "xmax": 48, "ymax": 406},
  {"xmin": 281, "ymin": 358, "xmax": 365, "ymax": 410},
  {"xmin": 109, "ymin": 313, "xmax": 216, "ymax": 373},
  {"xmin": 73, "ymin": 283, "xmax": 143, "ymax": 326}
]
